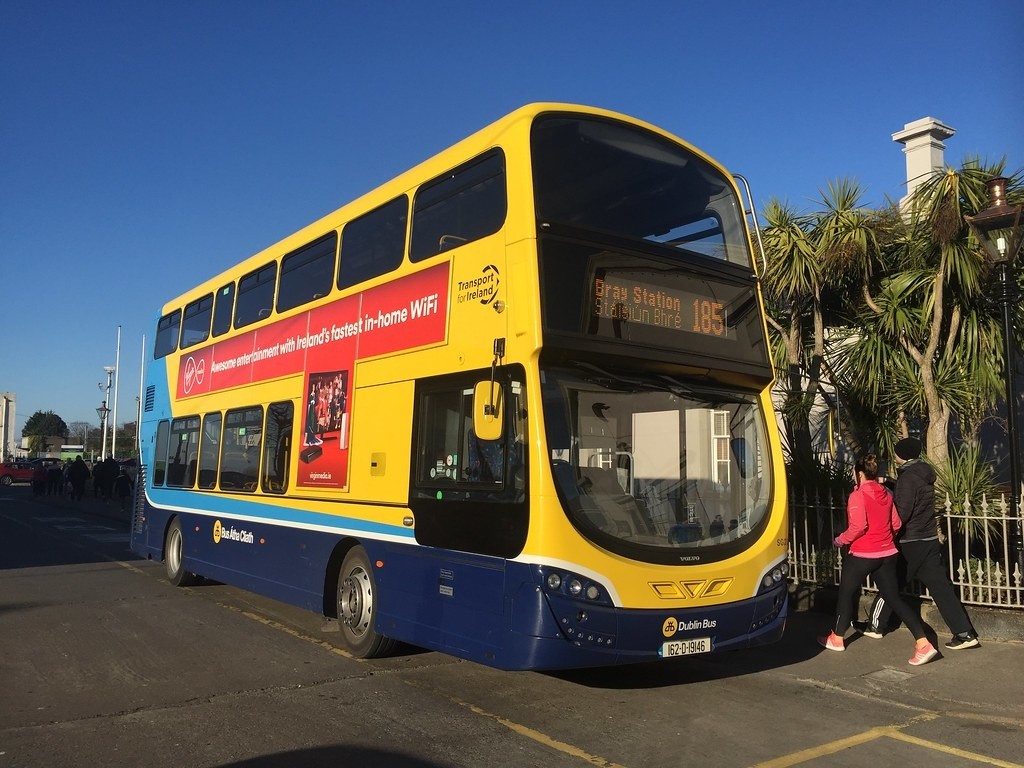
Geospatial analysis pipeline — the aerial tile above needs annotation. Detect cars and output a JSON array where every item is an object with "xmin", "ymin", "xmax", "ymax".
[
  {"xmin": 29, "ymin": 458, "xmax": 66, "ymax": 474},
  {"xmin": 0, "ymin": 462, "xmax": 49, "ymax": 486}
]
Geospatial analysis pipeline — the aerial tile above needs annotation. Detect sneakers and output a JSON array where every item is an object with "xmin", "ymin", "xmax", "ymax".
[
  {"xmin": 816, "ymin": 629, "xmax": 846, "ymax": 651},
  {"xmin": 945, "ymin": 636, "xmax": 979, "ymax": 650},
  {"xmin": 850, "ymin": 621, "xmax": 884, "ymax": 639},
  {"xmin": 908, "ymin": 643, "xmax": 942, "ymax": 665}
]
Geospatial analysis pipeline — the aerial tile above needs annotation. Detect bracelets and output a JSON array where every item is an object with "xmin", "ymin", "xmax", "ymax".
[{"xmin": 883, "ymin": 476, "xmax": 885, "ymax": 482}]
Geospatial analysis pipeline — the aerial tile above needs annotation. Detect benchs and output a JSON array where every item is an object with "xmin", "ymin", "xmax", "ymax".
[
  {"xmin": 439, "ymin": 235, "xmax": 468, "ymax": 251},
  {"xmin": 237, "ymin": 314, "xmax": 265, "ymax": 327}
]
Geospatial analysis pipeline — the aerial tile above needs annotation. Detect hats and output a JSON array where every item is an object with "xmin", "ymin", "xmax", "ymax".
[{"xmin": 894, "ymin": 437, "xmax": 923, "ymax": 461}]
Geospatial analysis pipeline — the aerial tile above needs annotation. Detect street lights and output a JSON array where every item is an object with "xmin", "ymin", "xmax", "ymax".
[{"xmin": 96, "ymin": 401, "xmax": 112, "ymax": 457}]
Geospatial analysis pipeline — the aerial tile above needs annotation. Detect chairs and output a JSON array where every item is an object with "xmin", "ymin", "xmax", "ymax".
[
  {"xmin": 222, "ymin": 452, "xmax": 248, "ymax": 486},
  {"xmin": 468, "ymin": 428, "xmax": 519, "ymax": 481},
  {"xmin": 184, "ymin": 450, "xmax": 216, "ymax": 485}
]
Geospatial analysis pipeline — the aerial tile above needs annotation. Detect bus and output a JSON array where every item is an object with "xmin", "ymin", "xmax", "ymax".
[{"xmin": 129, "ymin": 103, "xmax": 794, "ymax": 689}]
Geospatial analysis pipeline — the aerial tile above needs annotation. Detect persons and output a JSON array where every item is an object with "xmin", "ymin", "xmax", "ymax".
[
  {"xmin": 306, "ymin": 374, "xmax": 345, "ymax": 432},
  {"xmin": 34, "ymin": 452, "xmax": 134, "ymax": 512},
  {"xmin": 815, "ymin": 453, "xmax": 938, "ymax": 666},
  {"xmin": 850, "ymin": 438, "xmax": 979, "ymax": 650}
]
